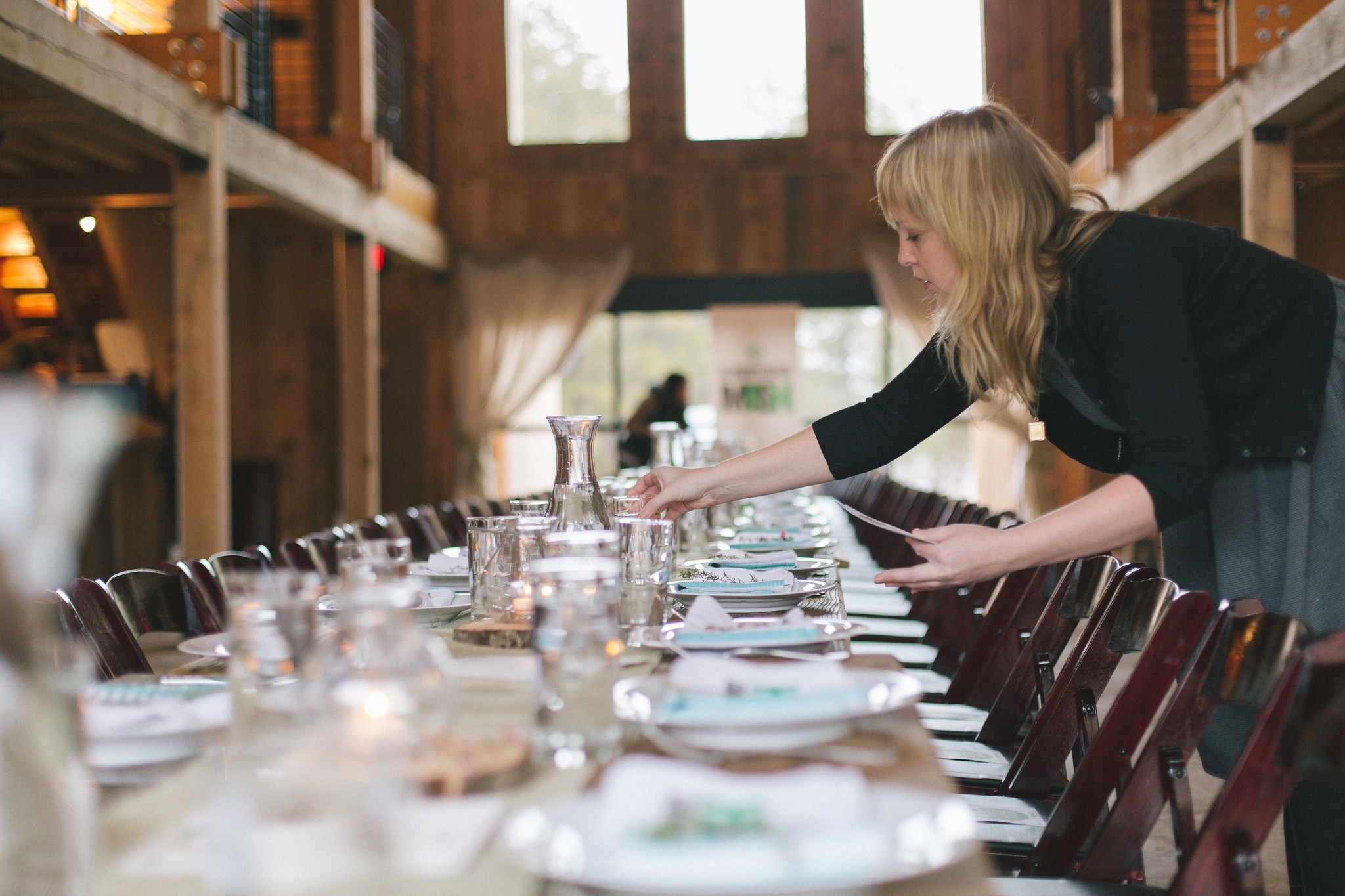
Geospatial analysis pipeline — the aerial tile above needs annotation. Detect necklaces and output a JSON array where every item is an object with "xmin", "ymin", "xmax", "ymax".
[{"xmin": 1028, "ymin": 332, "xmax": 1045, "ymax": 442}]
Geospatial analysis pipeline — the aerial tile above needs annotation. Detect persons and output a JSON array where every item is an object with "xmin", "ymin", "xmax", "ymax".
[
  {"xmin": 618, "ymin": 374, "xmax": 688, "ymax": 469},
  {"xmin": 621, "ymin": 101, "xmax": 1345, "ymax": 896}
]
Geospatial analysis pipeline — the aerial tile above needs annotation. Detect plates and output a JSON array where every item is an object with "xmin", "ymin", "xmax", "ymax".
[
  {"xmin": 72, "ymin": 515, "xmax": 555, "ymax": 781},
  {"xmin": 515, "ymin": 498, "xmax": 983, "ymax": 896}
]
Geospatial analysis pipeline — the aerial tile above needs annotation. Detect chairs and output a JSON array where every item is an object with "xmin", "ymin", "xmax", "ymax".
[
  {"xmin": 820, "ymin": 473, "xmax": 1345, "ymax": 896},
  {"xmin": 11, "ymin": 499, "xmax": 502, "ymax": 687}
]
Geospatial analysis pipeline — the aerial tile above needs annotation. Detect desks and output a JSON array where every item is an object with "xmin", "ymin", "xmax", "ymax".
[{"xmin": 90, "ymin": 578, "xmax": 992, "ymax": 896}]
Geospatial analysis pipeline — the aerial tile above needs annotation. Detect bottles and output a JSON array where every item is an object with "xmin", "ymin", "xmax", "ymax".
[
  {"xmin": 544, "ymin": 415, "xmax": 611, "ymax": 532},
  {"xmin": 0, "ymin": 381, "xmax": 121, "ymax": 896},
  {"xmin": 650, "ymin": 422, "xmax": 683, "ymax": 467}
]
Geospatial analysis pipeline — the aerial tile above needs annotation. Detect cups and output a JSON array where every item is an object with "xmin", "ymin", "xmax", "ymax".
[{"xmin": 218, "ymin": 498, "xmax": 678, "ymax": 863}]
